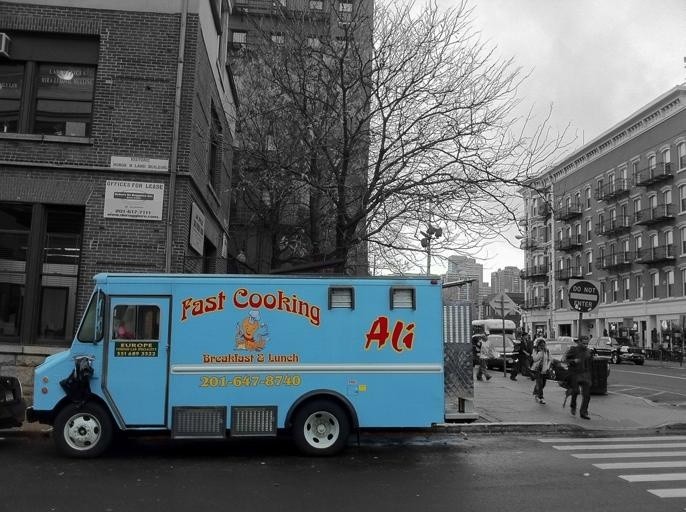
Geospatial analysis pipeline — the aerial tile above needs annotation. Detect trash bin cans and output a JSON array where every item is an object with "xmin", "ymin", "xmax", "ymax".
[{"xmin": 592, "ymin": 356, "xmax": 608, "ymax": 395}]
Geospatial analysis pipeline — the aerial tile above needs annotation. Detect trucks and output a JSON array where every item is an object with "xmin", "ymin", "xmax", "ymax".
[{"xmin": 25, "ymin": 272, "xmax": 444, "ymax": 457}]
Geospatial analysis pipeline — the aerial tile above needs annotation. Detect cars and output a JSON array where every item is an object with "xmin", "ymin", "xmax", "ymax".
[
  {"xmin": 545, "ymin": 341, "xmax": 572, "ymax": 380},
  {"xmin": 0, "ymin": 375, "xmax": 26, "ymax": 429}
]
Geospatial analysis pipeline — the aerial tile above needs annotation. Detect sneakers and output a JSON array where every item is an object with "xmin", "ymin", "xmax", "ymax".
[
  {"xmin": 541, "ymin": 398, "xmax": 546, "ymax": 404},
  {"xmin": 581, "ymin": 413, "xmax": 590, "ymax": 420},
  {"xmin": 477, "ymin": 376, "xmax": 492, "ymax": 380},
  {"xmin": 571, "ymin": 407, "xmax": 575, "ymax": 415}
]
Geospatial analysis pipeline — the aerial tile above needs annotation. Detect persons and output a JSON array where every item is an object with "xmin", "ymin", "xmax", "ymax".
[
  {"xmin": 531, "ymin": 337, "xmax": 552, "ymax": 404},
  {"xmin": 114, "ymin": 308, "xmax": 136, "ymax": 339},
  {"xmin": 510, "ymin": 332, "xmax": 535, "ymax": 382},
  {"xmin": 476, "ymin": 334, "xmax": 493, "ymax": 382},
  {"xmin": 566, "ymin": 335, "xmax": 602, "ymax": 420}
]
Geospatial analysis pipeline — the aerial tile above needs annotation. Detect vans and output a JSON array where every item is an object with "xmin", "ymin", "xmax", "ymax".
[{"xmin": 472, "ymin": 334, "xmax": 521, "ymax": 372}]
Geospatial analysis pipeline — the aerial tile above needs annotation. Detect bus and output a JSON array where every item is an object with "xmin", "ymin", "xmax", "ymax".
[{"xmin": 471, "ymin": 318, "xmax": 517, "ymax": 340}]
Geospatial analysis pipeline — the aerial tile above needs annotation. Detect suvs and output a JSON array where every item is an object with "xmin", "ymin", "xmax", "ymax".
[{"xmin": 587, "ymin": 337, "xmax": 647, "ymax": 365}]
